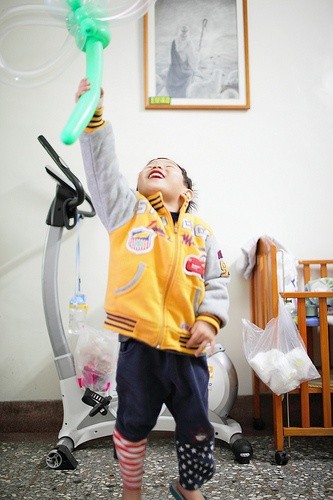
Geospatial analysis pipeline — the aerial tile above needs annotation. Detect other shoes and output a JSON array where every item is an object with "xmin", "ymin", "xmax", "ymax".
[{"xmin": 169, "ymin": 479, "xmax": 209, "ymax": 500}]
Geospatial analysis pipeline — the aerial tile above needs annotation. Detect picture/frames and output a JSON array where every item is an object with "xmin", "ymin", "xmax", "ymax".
[{"xmin": 141, "ymin": 0, "xmax": 251, "ymax": 111}]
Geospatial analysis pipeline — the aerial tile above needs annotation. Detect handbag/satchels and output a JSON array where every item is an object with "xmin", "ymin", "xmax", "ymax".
[
  {"xmin": 73, "ymin": 322, "xmax": 120, "ymax": 395},
  {"xmin": 241, "ymin": 294, "xmax": 320, "ymax": 396}
]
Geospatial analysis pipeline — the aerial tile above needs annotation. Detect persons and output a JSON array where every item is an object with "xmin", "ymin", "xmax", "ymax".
[{"xmin": 75, "ymin": 77, "xmax": 231, "ymax": 500}]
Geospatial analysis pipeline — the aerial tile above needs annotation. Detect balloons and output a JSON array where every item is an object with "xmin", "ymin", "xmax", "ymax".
[{"xmin": 0, "ymin": 0, "xmax": 156, "ymax": 145}]
